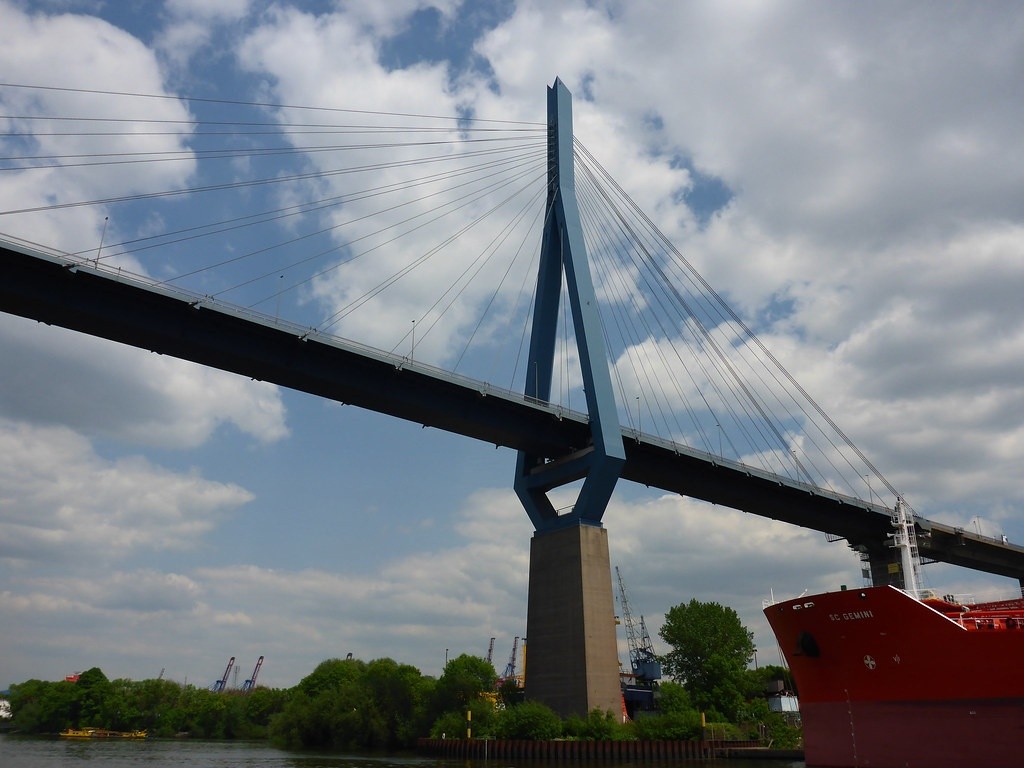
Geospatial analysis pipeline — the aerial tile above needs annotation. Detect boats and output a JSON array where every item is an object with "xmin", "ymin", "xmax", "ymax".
[
  {"xmin": 58, "ymin": 726, "xmax": 149, "ymax": 739},
  {"xmin": 760, "ymin": 491, "xmax": 1023, "ymax": 768}
]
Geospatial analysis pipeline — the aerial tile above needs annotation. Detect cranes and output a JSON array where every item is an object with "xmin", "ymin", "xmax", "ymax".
[{"xmin": 614, "ymin": 565, "xmax": 661, "ymax": 679}]
